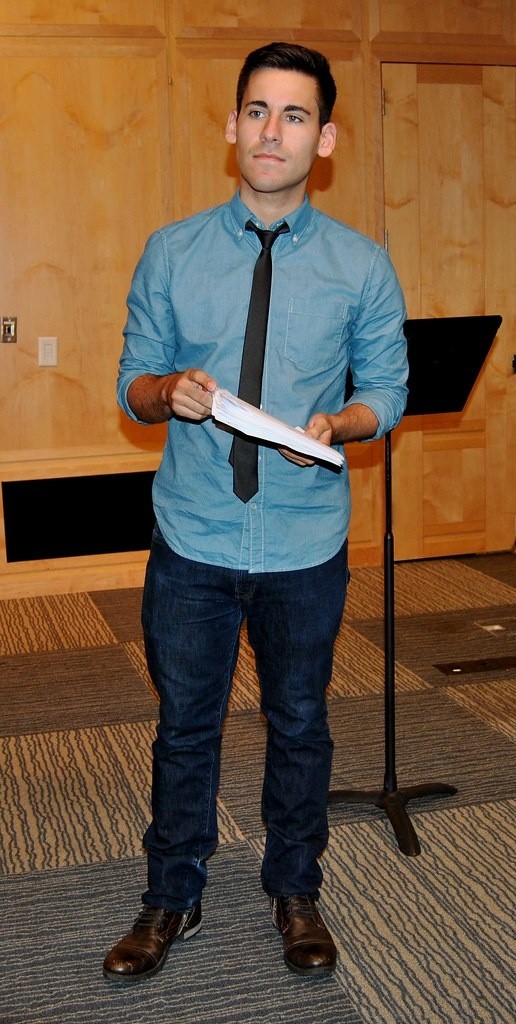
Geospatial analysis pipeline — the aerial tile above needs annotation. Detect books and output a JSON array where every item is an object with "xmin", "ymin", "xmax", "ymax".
[{"xmin": 208, "ymin": 388, "xmax": 344, "ymax": 468}]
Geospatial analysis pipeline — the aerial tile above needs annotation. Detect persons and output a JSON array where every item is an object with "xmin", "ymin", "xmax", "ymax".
[{"xmin": 96, "ymin": 40, "xmax": 409, "ymax": 983}]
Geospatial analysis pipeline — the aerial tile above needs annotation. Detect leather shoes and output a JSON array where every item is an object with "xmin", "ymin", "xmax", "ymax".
[
  {"xmin": 271, "ymin": 894, "xmax": 336, "ymax": 975},
  {"xmin": 103, "ymin": 900, "xmax": 203, "ymax": 982}
]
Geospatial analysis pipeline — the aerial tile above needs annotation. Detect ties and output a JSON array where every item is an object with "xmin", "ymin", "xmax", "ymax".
[{"xmin": 227, "ymin": 222, "xmax": 291, "ymax": 504}]
